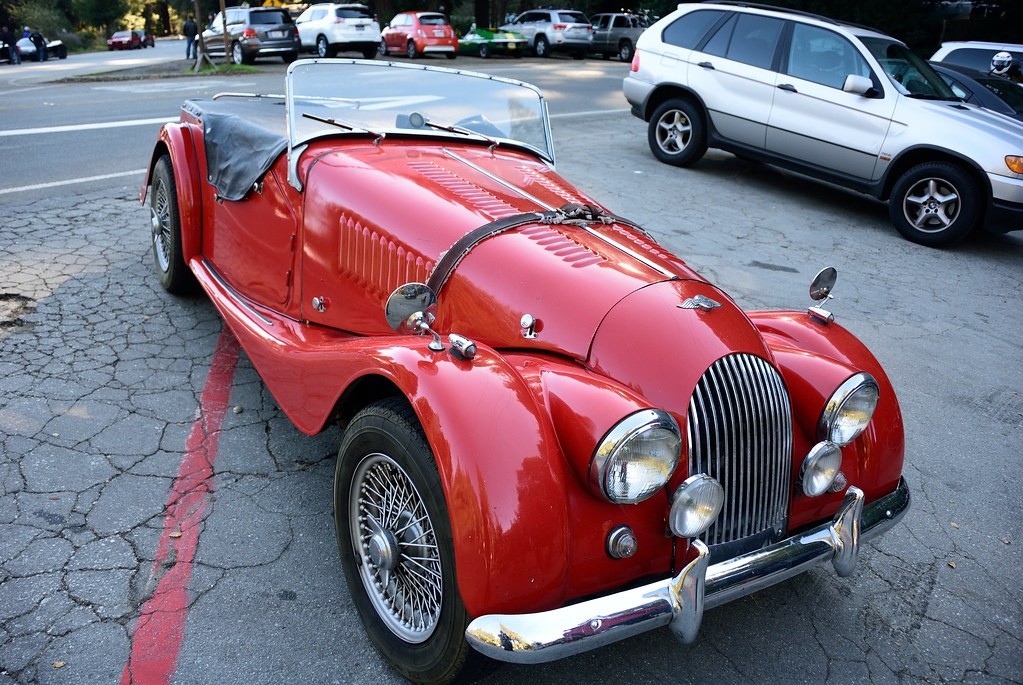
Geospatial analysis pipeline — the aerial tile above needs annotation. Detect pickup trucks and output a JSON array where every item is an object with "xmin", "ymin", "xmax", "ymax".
[{"xmin": 588, "ymin": 12, "xmax": 656, "ymax": 63}]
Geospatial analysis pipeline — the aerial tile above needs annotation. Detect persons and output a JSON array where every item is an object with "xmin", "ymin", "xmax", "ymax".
[
  {"xmin": 455, "ymin": 27, "xmax": 461, "ymax": 38},
  {"xmin": 2, "ymin": 26, "xmax": 22, "ymax": 64},
  {"xmin": 206, "ymin": 12, "xmax": 216, "ymax": 28},
  {"xmin": 29, "ymin": 26, "xmax": 47, "ymax": 63},
  {"xmin": 183, "ymin": 12, "xmax": 198, "ymax": 59},
  {"xmin": 439, "ymin": 5, "xmax": 450, "ymax": 21},
  {"xmin": 373, "ymin": 14, "xmax": 380, "ymax": 22},
  {"xmin": 505, "ymin": 13, "xmax": 517, "ymax": 24},
  {"xmin": 24, "ymin": 25, "xmax": 31, "ymax": 37}
]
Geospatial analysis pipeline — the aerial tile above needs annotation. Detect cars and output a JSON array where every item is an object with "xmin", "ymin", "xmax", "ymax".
[
  {"xmin": 496, "ymin": 9, "xmax": 595, "ymax": 60},
  {"xmin": 136, "ymin": 29, "xmax": 155, "ymax": 49},
  {"xmin": 905, "ymin": 40, "xmax": 1023, "ymax": 99},
  {"xmin": 14, "ymin": 37, "xmax": 53, "ymax": 62},
  {"xmin": 456, "ymin": 27, "xmax": 529, "ymax": 59},
  {"xmin": 381, "ymin": 10, "xmax": 458, "ymax": 60},
  {"xmin": 107, "ymin": 30, "xmax": 142, "ymax": 50},
  {"xmin": 141, "ymin": 59, "xmax": 912, "ymax": 685}
]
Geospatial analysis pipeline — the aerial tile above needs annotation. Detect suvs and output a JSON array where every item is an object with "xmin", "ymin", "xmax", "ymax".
[
  {"xmin": 194, "ymin": 7, "xmax": 301, "ymax": 66},
  {"xmin": 296, "ymin": 2, "xmax": 383, "ymax": 61},
  {"xmin": 622, "ymin": 3, "xmax": 1023, "ymax": 250}
]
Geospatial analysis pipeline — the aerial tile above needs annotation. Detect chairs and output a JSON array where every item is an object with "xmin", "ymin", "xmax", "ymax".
[
  {"xmin": 818, "ymin": 51, "xmax": 846, "ymax": 89},
  {"xmin": 741, "ymin": 36, "xmax": 772, "ymax": 67}
]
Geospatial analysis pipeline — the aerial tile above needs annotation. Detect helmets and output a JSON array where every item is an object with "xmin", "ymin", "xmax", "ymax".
[{"xmin": 991, "ymin": 53, "xmax": 1012, "ymax": 75}]
